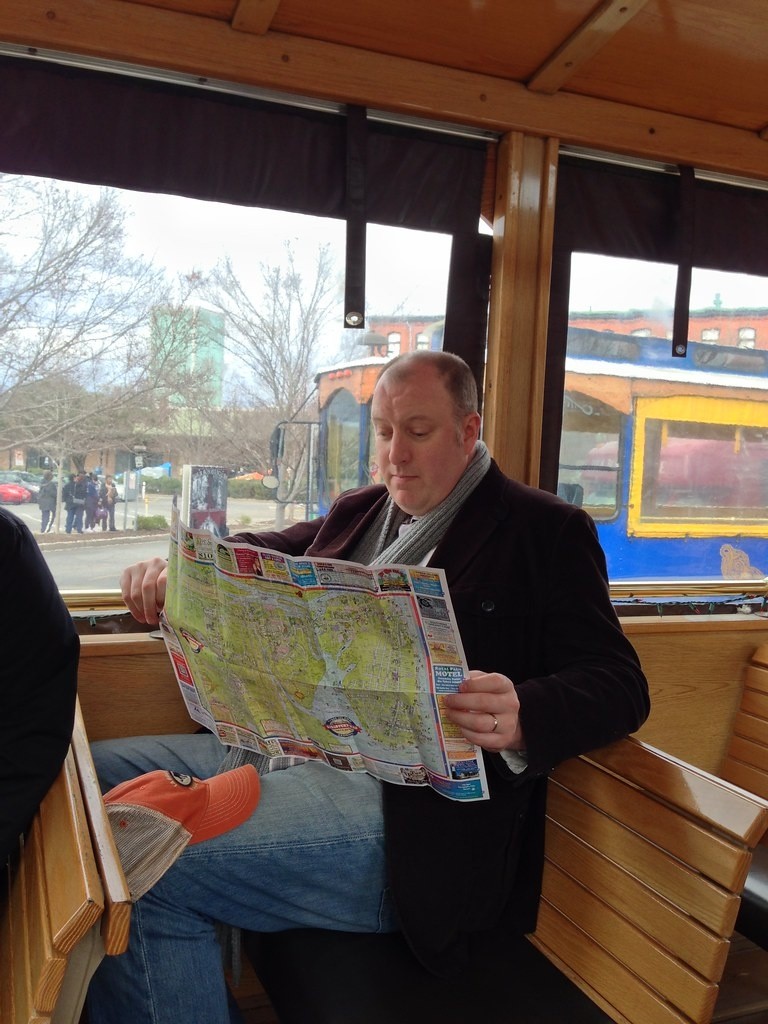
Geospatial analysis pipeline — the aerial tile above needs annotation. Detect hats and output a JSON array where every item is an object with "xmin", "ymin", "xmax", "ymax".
[{"xmin": 103, "ymin": 764, "xmax": 261, "ymax": 902}]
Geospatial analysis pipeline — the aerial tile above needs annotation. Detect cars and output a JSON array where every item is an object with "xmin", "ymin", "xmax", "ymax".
[{"xmin": 0, "ymin": 470, "xmax": 125, "ymax": 503}]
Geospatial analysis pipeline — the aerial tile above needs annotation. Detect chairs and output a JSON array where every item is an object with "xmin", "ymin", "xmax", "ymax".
[{"xmin": 558, "ymin": 483, "xmax": 584, "ymax": 510}]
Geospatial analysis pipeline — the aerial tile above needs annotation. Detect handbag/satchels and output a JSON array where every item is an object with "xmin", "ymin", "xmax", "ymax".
[
  {"xmin": 95, "ymin": 505, "xmax": 107, "ymax": 518},
  {"xmin": 72, "ymin": 497, "xmax": 84, "ymax": 505}
]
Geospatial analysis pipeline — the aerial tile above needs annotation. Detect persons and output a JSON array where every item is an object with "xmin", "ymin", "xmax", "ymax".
[
  {"xmin": 39, "ymin": 470, "xmax": 118, "ymax": 532},
  {"xmin": 87, "ymin": 351, "xmax": 651, "ymax": 1024},
  {"xmin": 0, "ymin": 506, "xmax": 81, "ymax": 848}
]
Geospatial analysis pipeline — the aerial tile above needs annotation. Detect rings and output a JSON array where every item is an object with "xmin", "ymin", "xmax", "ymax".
[{"xmin": 488, "ymin": 712, "xmax": 498, "ymax": 734}]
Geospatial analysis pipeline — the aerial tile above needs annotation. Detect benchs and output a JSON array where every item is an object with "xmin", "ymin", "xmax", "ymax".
[{"xmin": 1, "ymin": 615, "xmax": 768, "ymax": 1024}]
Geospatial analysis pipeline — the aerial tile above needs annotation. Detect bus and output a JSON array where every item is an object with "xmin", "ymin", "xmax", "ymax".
[{"xmin": 260, "ymin": 312, "xmax": 768, "ymax": 618}]
